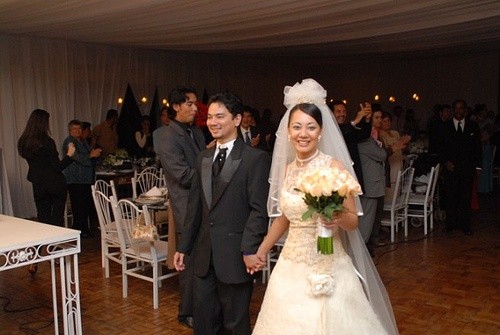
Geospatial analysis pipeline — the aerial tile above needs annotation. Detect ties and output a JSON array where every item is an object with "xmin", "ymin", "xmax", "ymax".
[
  {"xmin": 457, "ymin": 122, "xmax": 462, "ymax": 134},
  {"xmin": 213, "ymin": 147, "xmax": 228, "ymax": 176},
  {"xmin": 245, "ymin": 131, "xmax": 252, "ymax": 145}
]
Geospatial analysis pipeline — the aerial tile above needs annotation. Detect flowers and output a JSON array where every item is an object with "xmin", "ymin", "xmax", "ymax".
[{"xmin": 296, "ymin": 170, "xmax": 360, "ymax": 256}]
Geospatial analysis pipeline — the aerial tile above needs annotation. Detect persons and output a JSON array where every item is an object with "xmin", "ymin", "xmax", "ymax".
[
  {"xmin": 172, "ymin": 93, "xmax": 272, "ymax": 335},
  {"xmin": 330, "ymin": 97, "xmax": 500, "ymax": 246},
  {"xmin": 18, "ymin": 107, "xmax": 276, "ymax": 274},
  {"xmin": 153, "ymin": 86, "xmax": 205, "ymax": 329},
  {"xmin": 247, "ymin": 79, "xmax": 399, "ymax": 335}
]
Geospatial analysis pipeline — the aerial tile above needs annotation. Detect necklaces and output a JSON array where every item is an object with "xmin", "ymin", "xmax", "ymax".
[{"xmin": 295, "ymin": 149, "xmax": 318, "ymax": 165}]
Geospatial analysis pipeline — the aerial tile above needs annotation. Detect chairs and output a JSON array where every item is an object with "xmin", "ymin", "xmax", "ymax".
[
  {"xmin": 91, "ymin": 166, "xmax": 179, "ymax": 310},
  {"xmin": 260, "ymin": 217, "xmax": 288, "ymax": 284},
  {"xmin": 63, "ymin": 193, "xmax": 72, "ymax": 228},
  {"xmin": 381, "ymin": 166, "xmax": 414, "ymax": 242},
  {"xmin": 396, "ymin": 164, "xmax": 440, "ymax": 237}
]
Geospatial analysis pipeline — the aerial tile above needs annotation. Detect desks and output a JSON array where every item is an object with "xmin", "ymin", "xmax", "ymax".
[
  {"xmin": 122, "ymin": 195, "xmax": 169, "ymax": 229},
  {"xmin": 94, "ymin": 169, "xmax": 143, "ymax": 198},
  {"xmin": 0, "ymin": 213, "xmax": 83, "ymax": 335}
]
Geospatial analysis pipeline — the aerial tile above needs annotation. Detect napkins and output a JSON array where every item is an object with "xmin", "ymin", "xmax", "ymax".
[{"xmin": 141, "ymin": 187, "xmax": 168, "ymax": 197}]
